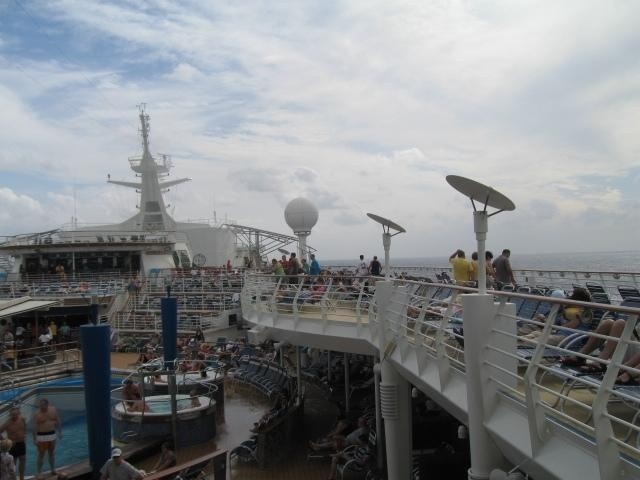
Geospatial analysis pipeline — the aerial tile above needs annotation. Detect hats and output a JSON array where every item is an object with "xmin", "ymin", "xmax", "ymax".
[{"xmin": 112, "ymin": 448, "xmax": 122, "ymax": 458}]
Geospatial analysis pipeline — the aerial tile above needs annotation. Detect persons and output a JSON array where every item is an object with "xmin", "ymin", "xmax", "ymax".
[
  {"xmin": 100, "ymin": 448, "xmax": 144, "ymax": 478},
  {"xmin": 272, "ymin": 252, "xmax": 331, "ymax": 304},
  {"xmin": 449, "ymin": 248, "xmax": 516, "ymax": 289},
  {"xmin": 32, "ymin": 398, "xmax": 62, "ymax": 478},
  {"xmin": 299, "ymin": 347, "xmax": 426, "ymax": 478},
  {"xmin": 9, "ymin": 263, "xmax": 89, "ymax": 297},
  {"xmin": 123, "ymin": 379, "xmax": 148, "ymax": 412},
  {"xmin": 149, "ymin": 442, "xmax": 176, "ymax": 478},
  {"xmin": 355, "ymin": 255, "xmax": 382, "ymax": 275},
  {"xmin": 452, "ymin": 287, "xmax": 639, "ymax": 385},
  {"xmin": 1, "ymin": 407, "xmax": 27, "ymax": 478},
  {"xmin": 137, "ymin": 334, "xmax": 238, "ymax": 371},
  {"xmin": 4, "ymin": 320, "xmax": 71, "ymax": 360}
]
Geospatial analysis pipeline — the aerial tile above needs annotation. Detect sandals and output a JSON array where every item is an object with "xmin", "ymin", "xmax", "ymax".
[{"xmin": 559, "ymin": 355, "xmax": 636, "ymax": 385}]
